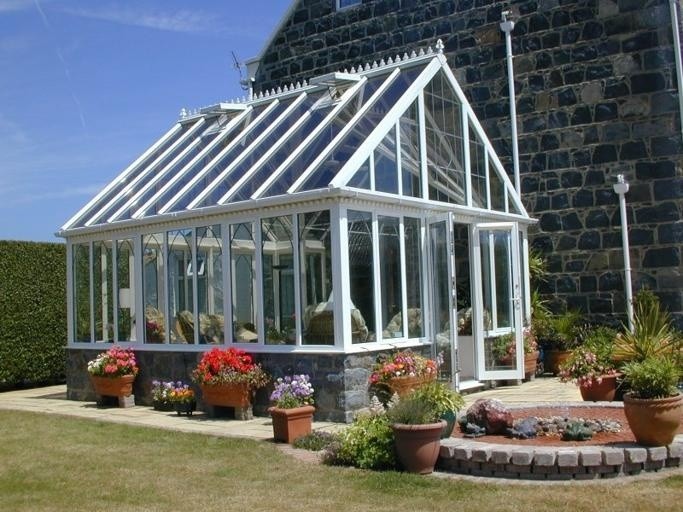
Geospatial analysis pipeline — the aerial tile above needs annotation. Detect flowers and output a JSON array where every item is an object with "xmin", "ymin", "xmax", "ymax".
[
  {"xmin": 169, "ymin": 379, "xmax": 196, "ymax": 403},
  {"xmin": 187, "ymin": 346, "xmax": 267, "ymax": 388},
  {"xmin": 154, "ymin": 380, "xmax": 173, "ymax": 402},
  {"xmin": 495, "ymin": 330, "xmax": 539, "ymax": 355},
  {"xmin": 264, "ymin": 373, "xmax": 318, "ymax": 408},
  {"xmin": 368, "ymin": 350, "xmax": 439, "ymax": 389},
  {"xmin": 559, "ymin": 343, "xmax": 616, "ymax": 386},
  {"xmin": 87, "ymin": 347, "xmax": 141, "ymax": 381}
]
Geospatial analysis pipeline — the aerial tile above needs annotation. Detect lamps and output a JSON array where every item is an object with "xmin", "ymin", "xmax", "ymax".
[{"xmin": 118, "ymin": 287, "xmax": 133, "ymax": 340}]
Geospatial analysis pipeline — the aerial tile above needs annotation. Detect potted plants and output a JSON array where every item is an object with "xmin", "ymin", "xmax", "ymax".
[
  {"xmin": 382, "ymin": 387, "xmax": 459, "ymax": 475},
  {"xmin": 613, "ymin": 302, "xmax": 683, "ymax": 446},
  {"xmin": 546, "ymin": 335, "xmax": 573, "ymax": 375}
]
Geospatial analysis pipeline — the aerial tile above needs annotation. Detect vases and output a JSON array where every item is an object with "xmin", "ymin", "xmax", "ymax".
[
  {"xmin": 507, "ymin": 350, "xmax": 540, "ymax": 372},
  {"xmin": 152, "ymin": 401, "xmax": 176, "ymax": 411},
  {"xmin": 579, "ymin": 371, "xmax": 622, "ymax": 402},
  {"xmin": 197, "ymin": 382, "xmax": 251, "ymax": 405},
  {"xmin": 268, "ymin": 405, "xmax": 315, "ymax": 447},
  {"xmin": 390, "ymin": 374, "xmax": 436, "ymax": 396},
  {"xmin": 172, "ymin": 401, "xmax": 196, "ymax": 417},
  {"xmin": 93, "ymin": 373, "xmax": 131, "ymax": 397}
]
